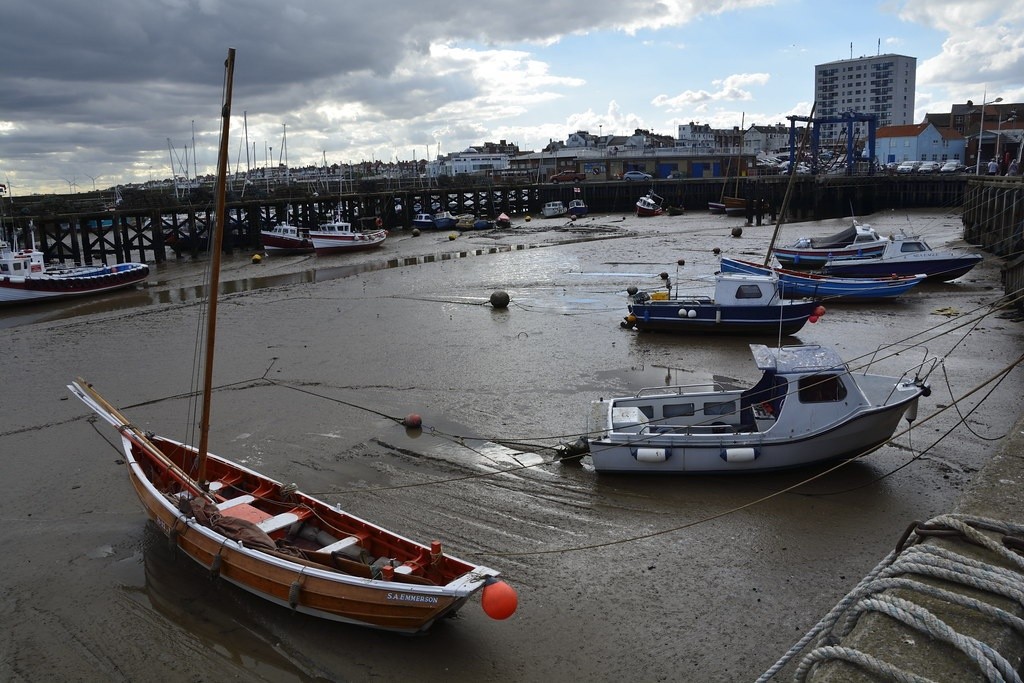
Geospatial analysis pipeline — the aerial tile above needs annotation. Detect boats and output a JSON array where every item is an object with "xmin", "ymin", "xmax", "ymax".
[
  {"xmin": 668, "ymin": 206, "xmax": 686, "ymax": 216},
  {"xmin": 496, "ymin": 212, "xmax": 512, "ymax": 228},
  {"xmin": 774, "ymin": 223, "xmax": 984, "ymax": 284},
  {"xmin": 0, "ymin": 218, "xmax": 150, "ymax": 300},
  {"xmin": 541, "ymin": 201, "xmax": 567, "ymax": 218},
  {"xmin": 413, "ymin": 213, "xmax": 434, "ymax": 230},
  {"xmin": 455, "ymin": 214, "xmax": 479, "ymax": 231},
  {"xmin": 635, "ymin": 188, "xmax": 664, "ymax": 216},
  {"xmin": 473, "ymin": 216, "xmax": 495, "ymax": 229},
  {"xmin": 582, "ymin": 342, "xmax": 935, "ymax": 483},
  {"xmin": 433, "ymin": 210, "xmax": 459, "ymax": 231},
  {"xmin": 567, "ymin": 187, "xmax": 588, "ymax": 216},
  {"xmin": 624, "ymin": 269, "xmax": 829, "ymax": 339},
  {"xmin": 258, "ymin": 192, "xmax": 315, "ymax": 255},
  {"xmin": 308, "ymin": 167, "xmax": 389, "ymax": 258}
]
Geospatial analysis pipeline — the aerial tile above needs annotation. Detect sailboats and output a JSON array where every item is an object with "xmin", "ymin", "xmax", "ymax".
[
  {"xmin": 708, "ymin": 111, "xmax": 769, "ymax": 215},
  {"xmin": 720, "ymin": 99, "xmax": 929, "ymax": 303},
  {"xmin": 65, "ymin": 45, "xmax": 499, "ymax": 637}
]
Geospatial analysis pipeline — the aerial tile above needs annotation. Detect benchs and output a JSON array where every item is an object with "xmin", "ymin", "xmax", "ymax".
[
  {"xmin": 315, "ymin": 537, "xmax": 358, "ymax": 554},
  {"xmin": 213, "ymin": 495, "xmax": 255, "ymax": 512},
  {"xmin": 174, "ymin": 482, "xmax": 223, "ymax": 502},
  {"xmin": 255, "ymin": 513, "xmax": 298, "ymax": 534},
  {"xmin": 393, "ymin": 565, "xmax": 412, "ymax": 575}
]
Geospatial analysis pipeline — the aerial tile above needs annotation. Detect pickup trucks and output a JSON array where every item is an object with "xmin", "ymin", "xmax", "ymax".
[{"xmin": 550, "ymin": 170, "xmax": 586, "ymax": 185}]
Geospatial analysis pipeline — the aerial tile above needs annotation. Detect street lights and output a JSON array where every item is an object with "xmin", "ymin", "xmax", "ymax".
[
  {"xmin": 996, "ymin": 117, "xmax": 1014, "ymax": 166},
  {"xmin": 976, "ymin": 96, "xmax": 1004, "ymax": 175}
]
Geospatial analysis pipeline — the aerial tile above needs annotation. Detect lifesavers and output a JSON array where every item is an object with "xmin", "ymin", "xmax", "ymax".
[
  {"xmin": 375, "ymin": 218, "xmax": 382, "ymax": 226},
  {"xmin": 20, "ymin": 248, "xmax": 38, "ymax": 253},
  {"xmin": 26, "ymin": 267, "xmax": 150, "ymax": 289}
]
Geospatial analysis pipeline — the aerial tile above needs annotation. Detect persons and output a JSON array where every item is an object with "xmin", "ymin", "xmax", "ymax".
[
  {"xmin": 1008, "ymin": 159, "xmax": 1018, "ymax": 176},
  {"xmin": 988, "ymin": 158, "xmax": 998, "ymax": 175}
]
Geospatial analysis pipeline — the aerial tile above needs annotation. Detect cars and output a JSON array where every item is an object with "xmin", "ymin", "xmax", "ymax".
[
  {"xmin": 888, "ymin": 160, "xmax": 946, "ymax": 173},
  {"xmin": 965, "ymin": 162, "xmax": 999, "ymax": 175},
  {"xmin": 941, "ymin": 161, "xmax": 968, "ymax": 174},
  {"xmin": 623, "ymin": 170, "xmax": 652, "ymax": 182},
  {"xmin": 757, "ymin": 150, "xmax": 862, "ymax": 174}
]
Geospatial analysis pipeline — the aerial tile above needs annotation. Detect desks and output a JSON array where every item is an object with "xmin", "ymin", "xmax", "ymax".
[{"xmin": 750, "ymin": 404, "xmax": 775, "ymax": 433}]
